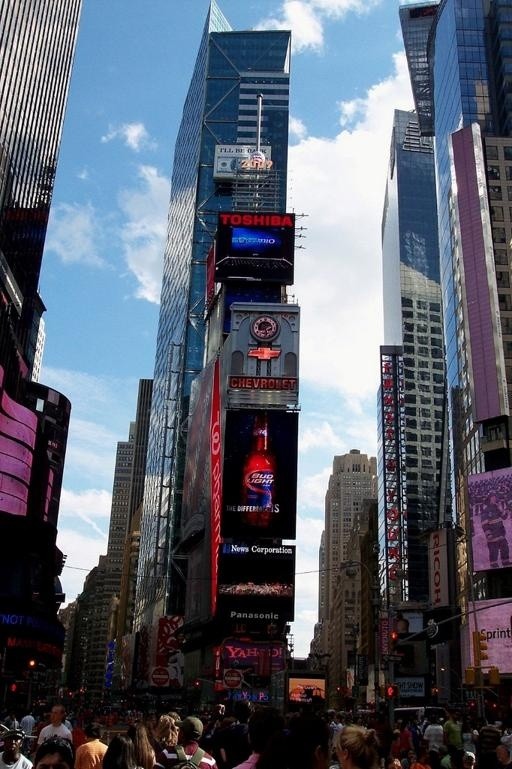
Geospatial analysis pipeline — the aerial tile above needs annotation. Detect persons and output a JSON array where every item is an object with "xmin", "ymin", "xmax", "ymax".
[
  {"xmin": 466, "ymin": 474, "xmax": 512, "ymax": 571},
  {"xmin": 0, "ymin": 688, "xmax": 512, "ymax": 769}
]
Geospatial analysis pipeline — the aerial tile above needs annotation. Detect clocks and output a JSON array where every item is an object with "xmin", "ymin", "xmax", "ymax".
[{"xmin": 250, "ymin": 317, "xmax": 280, "ymax": 344}]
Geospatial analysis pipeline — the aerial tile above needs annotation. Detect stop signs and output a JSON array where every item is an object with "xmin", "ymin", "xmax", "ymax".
[
  {"xmin": 223, "ymin": 667, "xmax": 243, "ymax": 689},
  {"xmin": 150, "ymin": 666, "xmax": 170, "ymax": 688}
]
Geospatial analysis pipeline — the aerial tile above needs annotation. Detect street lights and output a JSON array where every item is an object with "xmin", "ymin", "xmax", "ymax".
[
  {"xmin": 339, "ymin": 561, "xmax": 380, "ymax": 718},
  {"xmin": 439, "ymin": 666, "xmax": 463, "ymax": 703}
]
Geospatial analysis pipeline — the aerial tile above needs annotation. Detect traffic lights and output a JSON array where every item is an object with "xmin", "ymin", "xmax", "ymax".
[
  {"xmin": 193, "ymin": 680, "xmax": 203, "ymax": 688},
  {"xmin": 430, "ymin": 687, "xmax": 438, "ymax": 695},
  {"xmin": 336, "ymin": 685, "xmax": 342, "ymax": 692},
  {"xmin": 468, "ymin": 701, "xmax": 475, "ymax": 709},
  {"xmin": 472, "ymin": 631, "xmax": 488, "ymax": 661},
  {"xmin": 130, "ymin": 529, "xmax": 141, "ymax": 566},
  {"xmin": 390, "ymin": 631, "xmax": 398, "ymax": 652},
  {"xmin": 28, "ymin": 659, "xmax": 37, "ymax": 667},
  {"xmin": 491, "ymin": 703, "xmax": 497, "ymax": 712},
  {"xmin": 384, "ymin": 684, "xmax": 398, "ymax": 698},
  {"xmin": 9, "ymin": 683, "xmax": 17, "ymax": 692}
]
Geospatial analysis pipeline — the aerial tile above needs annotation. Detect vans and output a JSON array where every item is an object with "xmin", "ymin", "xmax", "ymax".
[{"xmin": 393, "ymin": 707, "xmax": 453, "ymax": 725}]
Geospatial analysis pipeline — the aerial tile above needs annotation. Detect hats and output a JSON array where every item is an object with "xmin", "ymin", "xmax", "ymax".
[{"xmin": 174, "ymin": 716, "xmax": 204, "ymax": 736}]
